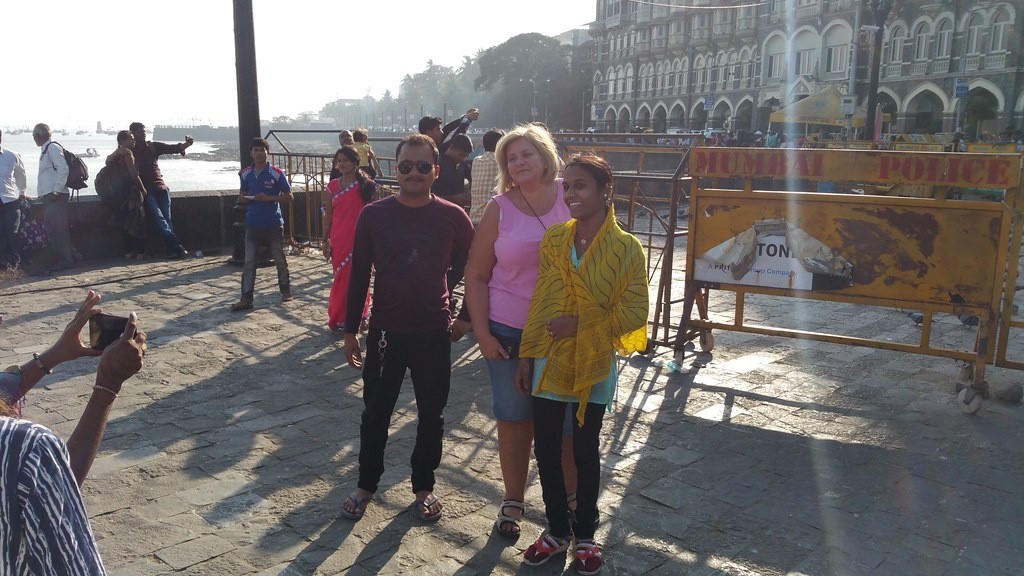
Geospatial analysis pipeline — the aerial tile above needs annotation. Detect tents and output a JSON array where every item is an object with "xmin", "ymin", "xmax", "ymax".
[{"xmin": 760, "ymin": 83, "xmax": 892, "ymax": 149}]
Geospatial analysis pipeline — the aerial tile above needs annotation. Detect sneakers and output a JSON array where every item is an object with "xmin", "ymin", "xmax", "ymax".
[
  {"xmin": 229, "ymin": 297, "xmax": 255, "ymax": 312},
  {"xmin": 167, "ymin": 233, "xmax": 189, "ymax": 259},
  {"xmin": 523, "ymin": 537, "xmax": 571, "ymax": 566},
  {"xmin": 280, "ymin": 287, "xmax": 293, "ymax": 301},
  {"xmin": 163, "ymin": 245, "xmax": 177, "ymax": 260},
  {"xmin": 573, "ymin": 543, "xmax": 605, "ymax": 575}
]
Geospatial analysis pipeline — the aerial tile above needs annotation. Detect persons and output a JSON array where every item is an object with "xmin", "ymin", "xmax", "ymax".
[
  {"xmin": 0, "ymin": 122, "xmax": 195, "ymax": 275},
  {"xmin": 0, "ymin": 290, "xmax": 148, "ymax": 489},
  {"xmin": 340, "ymin": 133, "xmax": 478, "ymax": 522},
  {"xmin": 319, "ymin": 146, "xmax": 380, "ymax": 335},
  {"xmin": 549, "ymin": 128, "xmax": 811, "ymax": 151},
  {"xmin": 326, "ymin": 108, "xmax": 507, "ymax": 320},
  {"xmin": 1, "ymin": 392, "xmax": 108, "ymax": 576},
  {"xmin": 512, "ymin": 148, "xmax": 651, "ymax": 576},
  {"xmin": 464, "ymin": 121, "xmax": 600, "ymax": 539},
  {"xmin": 229, "ymin": 136, "xmax": 295, "ymax": 312},
  {"xmin": 942, "ymin": 125, "xmax": 1023, "ymax": 153}
]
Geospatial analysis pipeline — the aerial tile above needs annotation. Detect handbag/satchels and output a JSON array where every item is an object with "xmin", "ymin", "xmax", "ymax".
[{"xmin": 17, "ymin": 195, "xmax": 52, "ymax": 251}]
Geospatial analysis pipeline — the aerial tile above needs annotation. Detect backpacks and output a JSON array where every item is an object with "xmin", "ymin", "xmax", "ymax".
[
  {"xmin": 94, "ymin": 153, "xmax": 136, "ymax": 206},
  {"xmin": 43, "ymin": 140, "xmax": 90, "ymax": 191}
]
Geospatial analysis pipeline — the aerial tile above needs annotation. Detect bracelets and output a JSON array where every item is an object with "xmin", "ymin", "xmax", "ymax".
[
  {"xmin": 91, "ymin": 384, "xmax": 118, "ymax": 399},
  {"xmin": 321, "ymin": 242, "xmax": 327, "ymax": 250},
  {"xmin": 33, "ymin": 352, "xmax": 53, "ymax": 375}
]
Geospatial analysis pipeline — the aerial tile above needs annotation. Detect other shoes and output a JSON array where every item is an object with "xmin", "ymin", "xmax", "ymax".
[
  {"xmin": 137, "ymin": 246, "xmax": 146, "ymax": 259},
  {"xmin": 124, "ymin": 245, "xmax": 137, "ymax": 258}
]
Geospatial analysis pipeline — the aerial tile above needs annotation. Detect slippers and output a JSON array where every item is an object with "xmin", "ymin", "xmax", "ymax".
[
  {"xmin": 413, "ymin": 493, "xmax": 444, "ymax": 521},
  {"xmin": 340, "ymin": 489, "xmax": 373, "ymax": 520}
]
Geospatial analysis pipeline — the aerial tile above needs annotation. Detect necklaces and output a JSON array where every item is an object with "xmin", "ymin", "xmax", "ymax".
[{"xmin": 575, "ymin": 226, "xmax": 601, "ymax": 244}]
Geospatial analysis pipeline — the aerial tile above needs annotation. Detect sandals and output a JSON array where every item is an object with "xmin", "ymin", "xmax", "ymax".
[
  {"xmin": 564, "ymin": 492, "xmax": 601, "ymax": 526},
  {"xmin": 496, "ymin": 496, "xmax": 525, "ymax": 537}
]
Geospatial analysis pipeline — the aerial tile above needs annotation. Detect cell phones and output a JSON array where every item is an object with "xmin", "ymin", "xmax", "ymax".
[
  {"xmin": 243, "ymin": 195, "xmax": 256, "ymax": 201},
  {"xmin": 185, "ymin": 135, "xmax": 190, "ymax": 141},
  {"xmin": 90, "ymin": 314, "xmax": 130, "ymax": 352}
]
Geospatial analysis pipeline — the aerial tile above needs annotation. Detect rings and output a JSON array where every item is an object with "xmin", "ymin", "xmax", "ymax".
[
  {"xmin": 140, "ymin": 348, "xmax": 146, "ymax": 356},
  {"xmin": 547, "ymin": 320, "xmax": 550, "ymax": 324},
  {"xmin": 550, "ymin": 331, "xmax": 554, "ymax": 336}
]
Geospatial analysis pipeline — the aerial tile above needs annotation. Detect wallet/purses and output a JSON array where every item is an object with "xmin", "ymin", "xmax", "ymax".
[{"xmin": 488, "ymin": 332, "xmax": 518, "ymax": 361}]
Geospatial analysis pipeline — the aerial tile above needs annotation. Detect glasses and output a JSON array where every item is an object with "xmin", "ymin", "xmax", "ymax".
[{"xmin": 394, "ymin": 160, "xmax": 440, "ymax": 177}]
